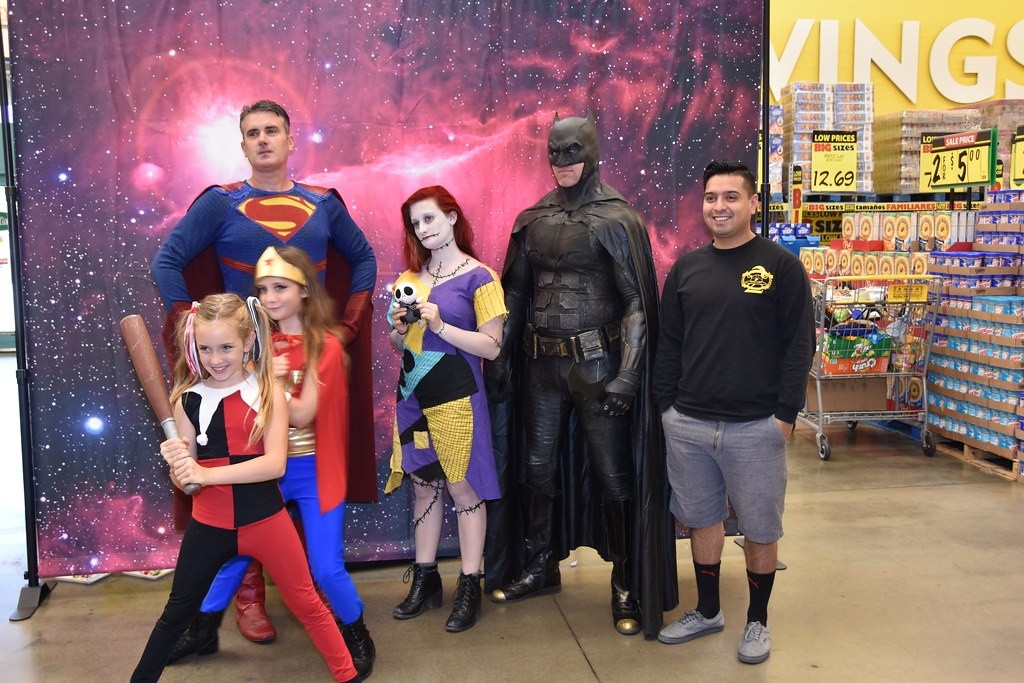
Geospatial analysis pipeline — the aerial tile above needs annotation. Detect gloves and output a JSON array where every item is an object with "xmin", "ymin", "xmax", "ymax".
[
  {"xmin": 484, "ymin": 363, "xmax": 513, "ymax": 402},
  {"xmin": 595, "ymin": 391, "xmax": 633, "ymax": 418}
]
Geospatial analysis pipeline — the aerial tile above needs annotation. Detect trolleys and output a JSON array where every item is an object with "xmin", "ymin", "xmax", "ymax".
[{"xmin": 793, "ymin": 273, "xmax": 943, "ymax": 460}]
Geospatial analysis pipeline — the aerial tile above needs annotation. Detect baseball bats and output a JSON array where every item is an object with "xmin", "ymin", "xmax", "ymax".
[{"xmin": 117, "ymin": 314, "xmax": 202, "ymax": 498}]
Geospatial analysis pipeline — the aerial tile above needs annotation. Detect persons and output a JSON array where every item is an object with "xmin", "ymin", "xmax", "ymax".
[
  {"xmin": 130, "ymin": 100, "xmax": 382, "ymax": 683},
  {"xmin": 490, "ymin": 108, "xmax": 680, "ymax": 638},
  {"xmin": 653, "ymin": 159, "xmax": 816, "ymax": 664},
  {"xmin": 376, "ymin": 186, "xmax": 510, "ymax": 631}
]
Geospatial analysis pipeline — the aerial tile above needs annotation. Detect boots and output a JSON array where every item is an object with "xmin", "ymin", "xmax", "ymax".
[
  {"xmin": 166, "ymin": 606, "xmax": 225, "ymax": 667},
  {"xmin": 491, "ymin": 463, "xmax": 565, "ymax": 603},
  {"xmin": 295, "ymin": 519, "xmax": 341, "ymax": 625},
  {"xmin": 601, "ymin": 495, "xmax": 641, "ymax": 635},
  {"xmin": 236, "ymin": 559, "xmax": 275, "ymax": 642},
  {"xmin": 445, "ymin": 571, "xmax": 482, "ymax": 632},
  {"xmin": 340, "ymin": 616, "xmax": 374, "ymax": 683},
  {"xmin": 392, "ymin": 556, "xmax": 443, "ymax": 618}
]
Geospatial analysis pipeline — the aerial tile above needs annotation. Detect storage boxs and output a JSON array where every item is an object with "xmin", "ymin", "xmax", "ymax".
[{"xmin": 756, "ymin": 83, "xmax": 1024, "ymax": 466}]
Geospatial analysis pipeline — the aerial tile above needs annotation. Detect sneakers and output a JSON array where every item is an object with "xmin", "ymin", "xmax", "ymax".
[
  {"xmin": 658, "ymin": 608, "xmax": 725, "ymax": 644},
  {"xmin": 738, "ymin": 622, "xmax": 772, "ymax": 663}
]
